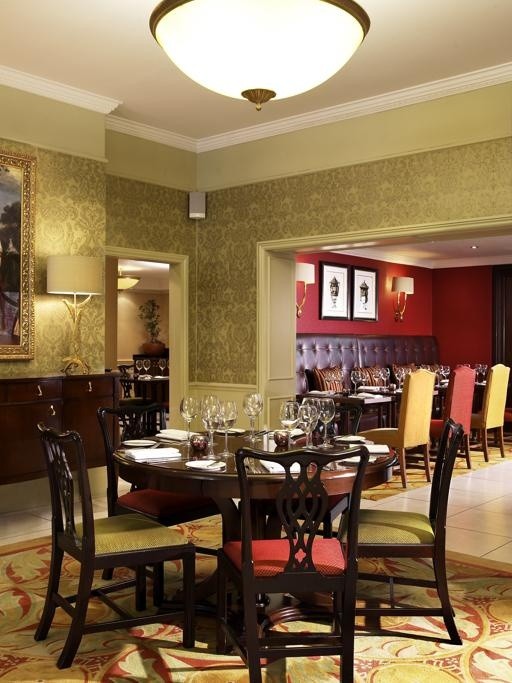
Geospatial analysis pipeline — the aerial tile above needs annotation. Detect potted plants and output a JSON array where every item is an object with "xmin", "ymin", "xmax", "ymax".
[{"xmin": 137, "ymin": 297, "xmax": 165, "ymax": 354}]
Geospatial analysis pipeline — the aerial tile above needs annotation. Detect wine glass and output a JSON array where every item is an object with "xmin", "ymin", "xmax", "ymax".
[
  {"xmin": 158, "ymin": 359, "xmax": 167, "ymax": 376},
  {"xmin": 180, "ymin": 393, "xmax": 336, "ymax": 456},
  {"xmin": 326, "ymin": 364, "xmax": 488, "ymax": 395},
  {"xmin": 143, "ymin": 360, "xmax": 151, "ymax": 377},
  {"xmin": 136, "ymin": 360, "xmax": 143, "ymax": 374}
]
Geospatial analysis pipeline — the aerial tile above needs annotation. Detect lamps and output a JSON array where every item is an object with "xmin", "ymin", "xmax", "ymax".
[
  {"xmin": 391, "ymin": 277, "xmax": 414, "ymax": 322},
  {"xmin": 149, "ymin": 0, "xmax": 370, "ymax": 111},
  {"xmin": 296, "ymin": 262, "xmax": 315, "ymax": 317},
  {"xmin": 46, "ymin": 255, "xmax": 103, "ymax": 374},
  {"xmin": 117, "ymin": 267, "xmax": 141, "ymax": 291}
]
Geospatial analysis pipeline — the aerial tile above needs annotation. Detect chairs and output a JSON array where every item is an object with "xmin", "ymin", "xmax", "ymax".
[
  {"xmin": 336, "ymin": 417, "xmax": 465, "ymax": 645},
  {"xmin": 296, "ymin": 333, "xmax": 512, "ymax": 501},
  {"xmin": 217, "ymin": 446, "xmax": 370, "ymax": 683},
  {"xmin": 34, "ymin": 422, "xmax": 195, "ymax": 669}
]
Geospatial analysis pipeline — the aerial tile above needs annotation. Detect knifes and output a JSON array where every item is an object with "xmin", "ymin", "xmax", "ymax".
[
  {"xmin": 150, "ymin": 441, "xmax": 163, "ymax": 449},
  {"xmin": 207, "ymin": 457, "xmax": 225, "ymax": 468}
]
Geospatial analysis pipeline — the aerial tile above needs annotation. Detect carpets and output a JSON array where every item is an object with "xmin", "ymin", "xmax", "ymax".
[{"xmin": 0, "ymin": 515, "xmax": 512, "ymax": 683}]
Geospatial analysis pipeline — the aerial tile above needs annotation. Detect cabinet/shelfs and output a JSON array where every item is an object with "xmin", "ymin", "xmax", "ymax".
[{"xmin": 0, "ymin": 374, "xmax": 120, "ymax": 485}]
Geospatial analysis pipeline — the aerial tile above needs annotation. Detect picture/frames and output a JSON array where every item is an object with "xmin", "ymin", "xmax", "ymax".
[
  {"xmin": 319, "ymin": 261, "xmax": 379, "ymax": 322},
  {"xmin": 0, "ymin": 149, "xmax": 37, "ymax": 361}
]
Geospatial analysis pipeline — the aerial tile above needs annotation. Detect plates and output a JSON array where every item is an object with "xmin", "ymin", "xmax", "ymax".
[
  {"xmin": 123, "ymin": 440, "xmax": 156, "ymax": 447},
  {"xmin": 185, "ymin": 460, "xmax": 226, "ymax": 470},
  {"xmin": 333, "ymin": 436, "xmax": 366, "ymax": 442}
]
